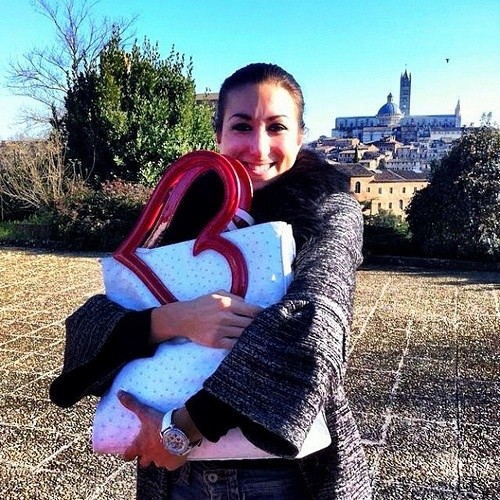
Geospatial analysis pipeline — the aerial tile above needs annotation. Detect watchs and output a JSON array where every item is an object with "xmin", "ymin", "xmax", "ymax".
[{"xmin": 159, "ymin": 407, "xmax": 203, "ymax": 458}]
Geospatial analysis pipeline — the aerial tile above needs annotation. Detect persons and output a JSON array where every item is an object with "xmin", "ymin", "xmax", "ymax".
[{"xmin": 50, "ymin": 62, "xmax": 373, "ymax": 500}]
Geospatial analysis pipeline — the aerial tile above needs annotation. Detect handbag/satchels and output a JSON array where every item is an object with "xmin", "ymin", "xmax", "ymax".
[{"xmin": 88, "ymin": 151, "xmax": 331, "ymax": 460}]
